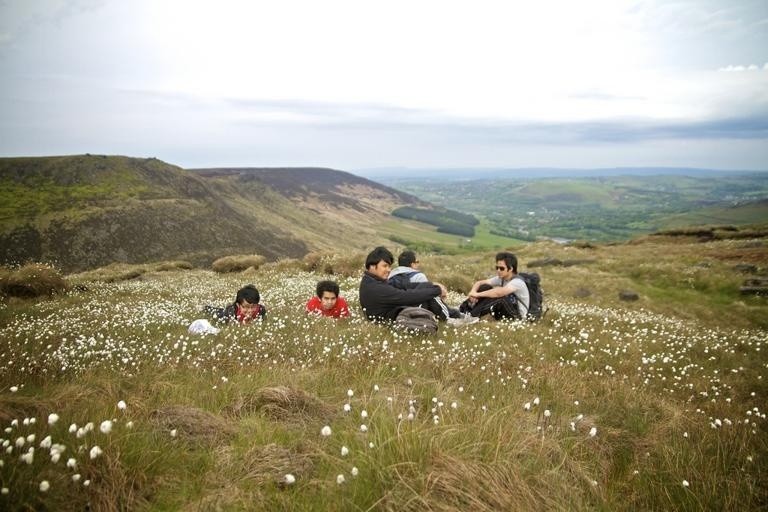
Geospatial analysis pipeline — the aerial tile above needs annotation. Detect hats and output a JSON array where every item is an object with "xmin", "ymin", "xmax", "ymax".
[{"xmin": 188, "ymin": 319, "xmax": 220, "ymax": 334}]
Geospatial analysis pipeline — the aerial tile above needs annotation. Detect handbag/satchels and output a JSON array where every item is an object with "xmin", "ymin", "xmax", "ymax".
[{"xmin": 396, "ymin": 307, "xmax": 438, "ymax": 334}]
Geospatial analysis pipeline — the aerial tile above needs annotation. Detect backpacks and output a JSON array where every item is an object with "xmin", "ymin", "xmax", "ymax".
[
  {"xmin": 511, "ymin": 272, "xmax": 542, "ymax": 318},
  {"xmin": 386, "ymin": 272, "xmax": 419, "ymax": 289}
]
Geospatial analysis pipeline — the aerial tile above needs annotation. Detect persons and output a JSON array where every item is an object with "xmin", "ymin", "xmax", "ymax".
[
  {"xmin": 359, "ymin": 247, "xmax": 480, "ymax": 326},
  {"xmin": 205, "ymin": 287, "xmax": 266, "ymax": 324},
  {"xmin": 460, "ymin": 252, "xmax": 530, "ymax": 319},
  {"xmin": 305, "ymin": 281, "xmax": 349, "ymax": 319},
  {"xmin": 388, "ymin": 251, "xmax": 428, "ymax": 284}
]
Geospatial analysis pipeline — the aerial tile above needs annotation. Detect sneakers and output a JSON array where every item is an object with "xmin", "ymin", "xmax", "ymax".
[{"xmin": 446, "ymin": 312, "xmax": 479, "ymax": 326}]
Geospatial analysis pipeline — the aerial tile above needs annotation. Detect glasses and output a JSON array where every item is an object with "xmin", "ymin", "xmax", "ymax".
[
  {"xmin": 496, "ymin": 266, "xmax": 504, "ymax": 271},
  {"xmin": 240, "ymin": 304, "xmax": 257, "ymax": 309}
]
